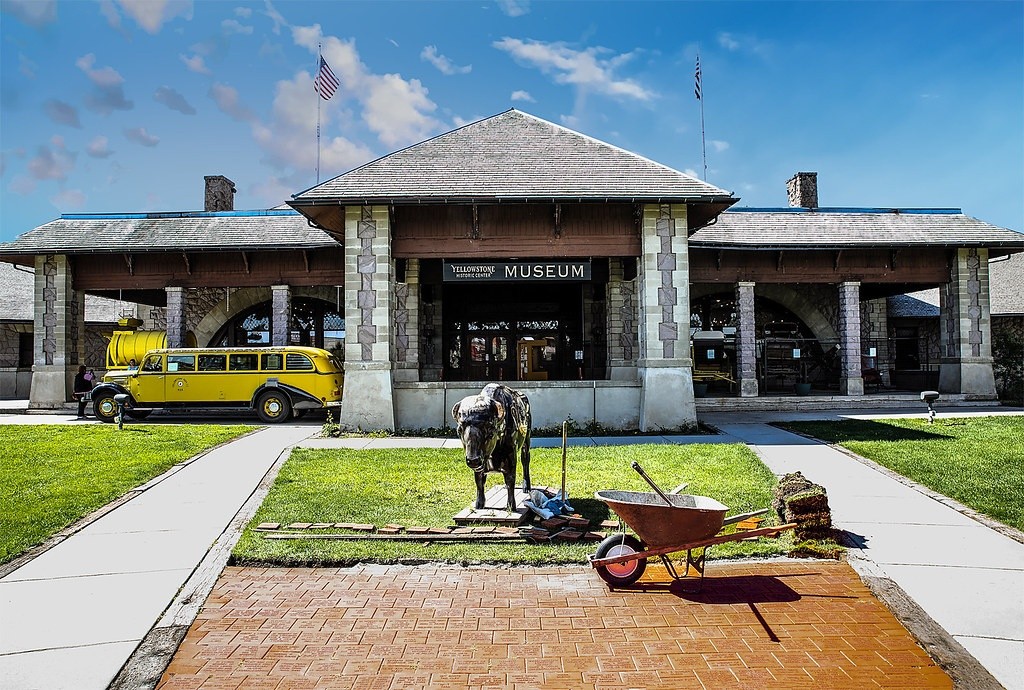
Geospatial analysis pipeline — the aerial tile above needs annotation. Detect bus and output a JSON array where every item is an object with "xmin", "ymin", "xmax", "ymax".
[{"xmin": 89, "ymin": 345, "xmax": 344, "ymax": 424}]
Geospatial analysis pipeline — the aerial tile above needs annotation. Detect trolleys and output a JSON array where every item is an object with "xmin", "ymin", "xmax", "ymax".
[{"xmin": 584, "ymin": 489, "xmax": 798, "ymax": 590}]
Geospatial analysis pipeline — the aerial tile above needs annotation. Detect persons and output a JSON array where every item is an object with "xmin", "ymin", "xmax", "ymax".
[
  {"xmin": 127, "ymin": 359, "xmax": 139, "ymax": 370},
  {"xmin": 74, "ymin": 364, "xmax": 96, "ymax": 420}
]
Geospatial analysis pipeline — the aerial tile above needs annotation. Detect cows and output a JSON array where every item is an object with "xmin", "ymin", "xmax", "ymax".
[{"xmin": 450, "ymin": 380, "xmax": 534, "ymax": 514}]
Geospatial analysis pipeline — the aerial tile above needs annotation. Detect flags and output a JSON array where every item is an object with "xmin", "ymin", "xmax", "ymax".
[
  {"xmin": 313, "ymin": 53, "xmax": 341, "ymax": 101},
  {"xmin": 695, "ymin": 54, "xmax": 701, "ymax": 101}
]
